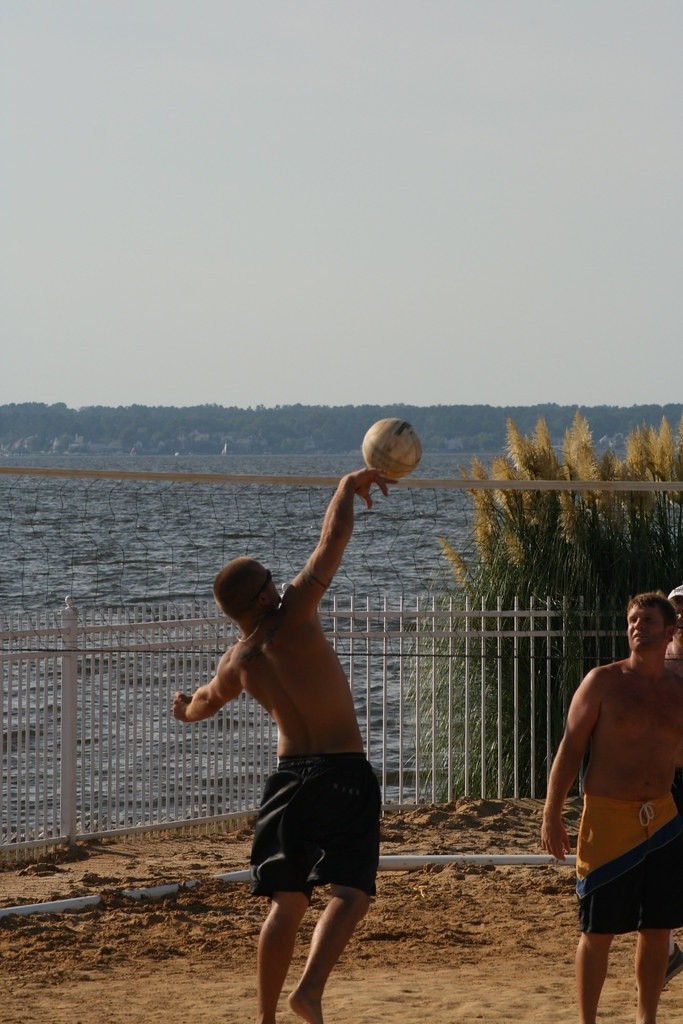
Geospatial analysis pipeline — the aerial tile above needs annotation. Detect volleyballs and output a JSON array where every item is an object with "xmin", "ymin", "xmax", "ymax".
[{"xmin": 362, "ymin": 417, "xmax": 421, "ymax": 479}]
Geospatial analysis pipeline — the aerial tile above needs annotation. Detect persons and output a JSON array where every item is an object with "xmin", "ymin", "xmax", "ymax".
[
  {"xmin": 173, "ymin": 469, "xmax": 400, "ymax": 1024},
  {"xmin": 540, "ymin": 593, "xmax": 683, "ymax": 1024},
  {"xmin": 660, "ymin": 585, "xmax": 683, "ymax": 988}
]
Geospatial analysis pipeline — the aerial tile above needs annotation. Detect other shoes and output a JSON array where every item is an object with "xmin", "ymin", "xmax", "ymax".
[{"xmin": 663, "ymin": 943, "xmax": 683, "ymax": 987}]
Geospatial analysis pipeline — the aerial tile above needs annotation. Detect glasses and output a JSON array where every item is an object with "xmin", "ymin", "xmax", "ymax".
[{"xmin": 253, "ymin": 570, "xmax": 272, "ymax": 601}]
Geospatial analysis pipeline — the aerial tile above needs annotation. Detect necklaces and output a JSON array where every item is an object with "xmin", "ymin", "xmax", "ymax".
[{"xmin": 236, "ymin": 622, "xmax": 261, "ymax": 643}]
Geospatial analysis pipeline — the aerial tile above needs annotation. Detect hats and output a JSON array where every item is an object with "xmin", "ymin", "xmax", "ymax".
[{"xmin": 667, "ymin": 585, "xmax": 683, "ymax": 601}]
